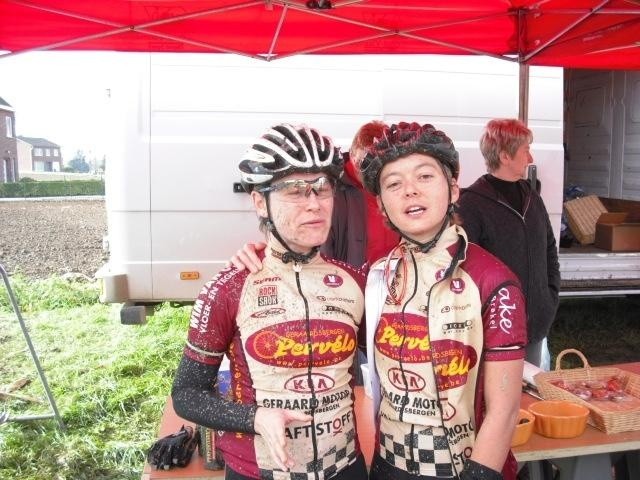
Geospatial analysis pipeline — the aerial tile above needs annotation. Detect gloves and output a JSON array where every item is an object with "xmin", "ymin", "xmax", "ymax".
[{"xmin": 145, "ymin": 424, "xmax": 199, "ymax": 471}]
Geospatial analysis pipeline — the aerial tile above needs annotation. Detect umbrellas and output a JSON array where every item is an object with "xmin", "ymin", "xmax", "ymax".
[{"xmin": 0, "ymin": 0, "xmax": 640, "ymax": 116}]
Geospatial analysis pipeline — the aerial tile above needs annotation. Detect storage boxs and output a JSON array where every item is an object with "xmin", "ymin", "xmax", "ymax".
[
  {"xmin": 562, "ymin": 194, "xmax": 608, "ymax": 246},
  {"xmin": 593, "ymin": 211, "xmax": 640, "ymax": 252}
]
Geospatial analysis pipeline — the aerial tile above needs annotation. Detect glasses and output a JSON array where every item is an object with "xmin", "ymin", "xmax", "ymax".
[{"xmin": 257, "ymin": 177, "xmax": 330, "ymax": 197}]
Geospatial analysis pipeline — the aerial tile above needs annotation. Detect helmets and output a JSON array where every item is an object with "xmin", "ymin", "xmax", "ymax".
[
  {"xmin": 361, "ymin": 122, "xmax": 460, "ymax": 254},
  {"xmin": 237, "ymin": 124, "xmax": 347, "ymax": 267}
]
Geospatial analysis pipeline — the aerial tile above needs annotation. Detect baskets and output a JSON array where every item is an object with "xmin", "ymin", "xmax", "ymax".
[{"xmin": 532, "ymin": 347, "xmax": 639, "ymax": 436}]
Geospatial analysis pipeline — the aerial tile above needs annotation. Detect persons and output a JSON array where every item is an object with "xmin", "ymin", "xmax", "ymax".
[
  {"xmin": 320, "ymin": 123, "xmax": 401, "ymax": 266},
  {"xmin": 225, "ymin": 122, "xmax": 527, "ymax": 480},
  {"xmin": 172, "ymin": 122, "xmax": 372, "ymax": 480},
  {"xmin": 456, "ymin": 118, "xmax": 560, "ymax": 384}
]
